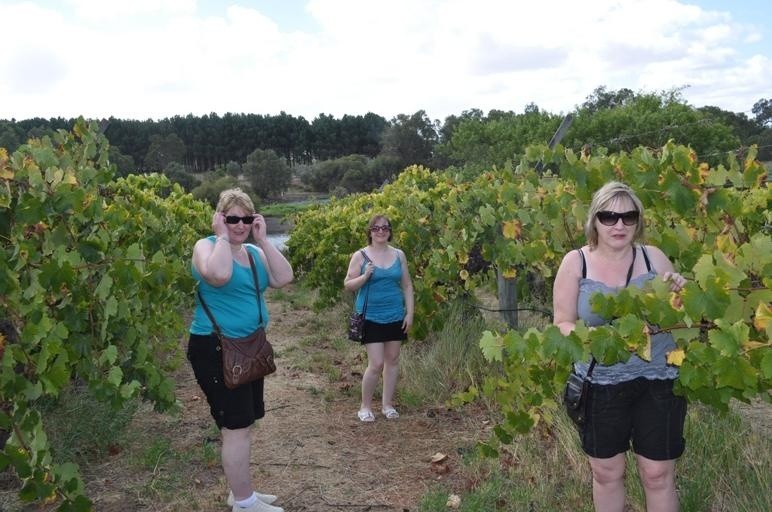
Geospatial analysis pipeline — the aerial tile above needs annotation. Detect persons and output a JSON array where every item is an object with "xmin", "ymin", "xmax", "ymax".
[
  {"xmin": 344, "ymin": 215, "xmax": 415, "ymax": 422},
  {"xmin": 553, "ymin": 181, "xmax": 686, "ymax": 511},
  {"xmin": 185, "ymin": 188, "xmax": 294, "ymax": 512}
]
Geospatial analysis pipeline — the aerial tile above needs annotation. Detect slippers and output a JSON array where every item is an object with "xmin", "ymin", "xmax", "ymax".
[
  {"xmin": 382, "ymin": 407, "xmax": 399, "ymax": 418},
  {"xmin": 358, "ymin": 410, "xmax": 375, "ymax": 422}
]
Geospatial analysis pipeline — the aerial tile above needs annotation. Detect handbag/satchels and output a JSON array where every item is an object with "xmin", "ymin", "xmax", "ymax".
[
  {"xmin": 220, "ymin": 328, "xmax": 276, "ymax": 388},
  {"xmin": 564, "ymin": 372, "xmax": 591, "ymax": 432},
  {"xmin": 349, "ymin": 313, "xmax": 365, "ymax": 341}
]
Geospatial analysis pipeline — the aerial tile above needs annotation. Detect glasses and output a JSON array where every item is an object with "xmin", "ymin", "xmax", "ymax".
[
  {"xmin": 369, "ymin": 226, "xmax": 392, "ymax": 232},
  {"xmin": 597, "ymin": 211, "xmax": 639, "ymax": 226},
  {"xmin": 222, "ymin": 215, "xmax": 255, "ymax": 224}
]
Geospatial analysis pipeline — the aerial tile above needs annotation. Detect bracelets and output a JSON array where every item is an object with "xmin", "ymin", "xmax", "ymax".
[{"xmin": 216, "ymin": 235, "xmax": 228, "ymax": 242}]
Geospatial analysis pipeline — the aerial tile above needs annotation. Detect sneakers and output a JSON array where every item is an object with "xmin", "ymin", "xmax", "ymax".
[
  {"xmin": 227, "ymin": 489, "xmax": 277, "ymax": 506},
  {"xmin": 232, "ymin": 500, "xmax": 284, "ymax": 512}
]
